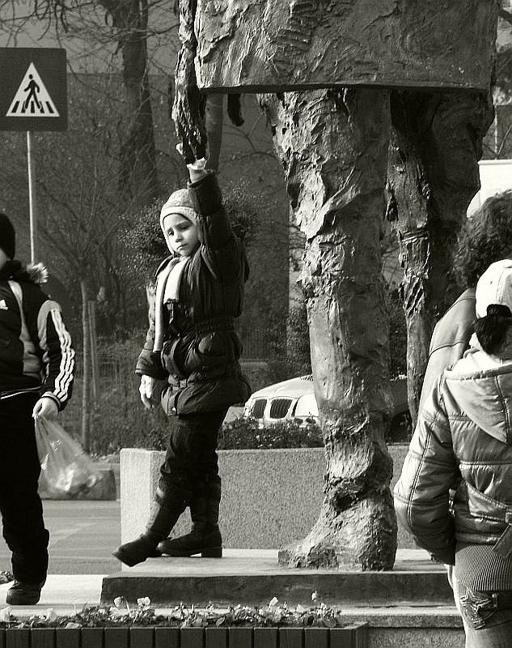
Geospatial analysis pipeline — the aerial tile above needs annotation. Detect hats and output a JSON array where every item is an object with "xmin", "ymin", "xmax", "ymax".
[
  {"xmin": 475, "ymin": 258, "xmax": 512, "ymax": 320},
  {"xmin": 0, "ymin": 215, "xmax": 17, "ymax": 259},
  {"xmin": 158, "ymin": 189, "xmax": 202, "ymax": 253}
]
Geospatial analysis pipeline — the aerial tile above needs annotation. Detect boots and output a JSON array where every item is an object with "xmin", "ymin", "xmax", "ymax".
[{"xmin": 112, "ymin": 476, "xmax": 223, "ymax": 565}]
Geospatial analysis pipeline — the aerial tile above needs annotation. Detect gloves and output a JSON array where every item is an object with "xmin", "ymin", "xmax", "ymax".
[
  {"xmin": 137, "ymin": 375, "xmax": 160, "ymax": 409},
  {"xmin": 176, "ymin": 136, "xmax": 206, "ymax": 173}
]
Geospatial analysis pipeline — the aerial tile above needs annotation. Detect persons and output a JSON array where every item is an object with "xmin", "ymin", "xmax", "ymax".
[
  {"xmin": 415, "ymin": 189, "xmax": 512, "ymax": 426},
  {"xmin": 392, "ymin": 258, "xmax": 511, "ymax": 648},
  {"xmin": 111, "ymin": 136, "xmax": 250, "ymax": 567},
  {"xmin": 0, "ymin": 213, "xmax": 78, "ymax": 606}
]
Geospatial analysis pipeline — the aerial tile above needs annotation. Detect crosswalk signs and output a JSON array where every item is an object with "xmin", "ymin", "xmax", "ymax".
[{"xmin": 0, "ymin": 49, "xmax": 68, "ymax": 132}]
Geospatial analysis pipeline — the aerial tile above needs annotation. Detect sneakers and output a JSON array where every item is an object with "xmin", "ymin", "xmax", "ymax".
[{"xmin": 6, "ymin": 572, "xmax": 47, "ymax": 603}]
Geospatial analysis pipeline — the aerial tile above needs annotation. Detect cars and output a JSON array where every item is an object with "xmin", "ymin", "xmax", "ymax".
[{"xmin": 244, "ymin": 369, "xmax": 413, "ymax": 437}]
